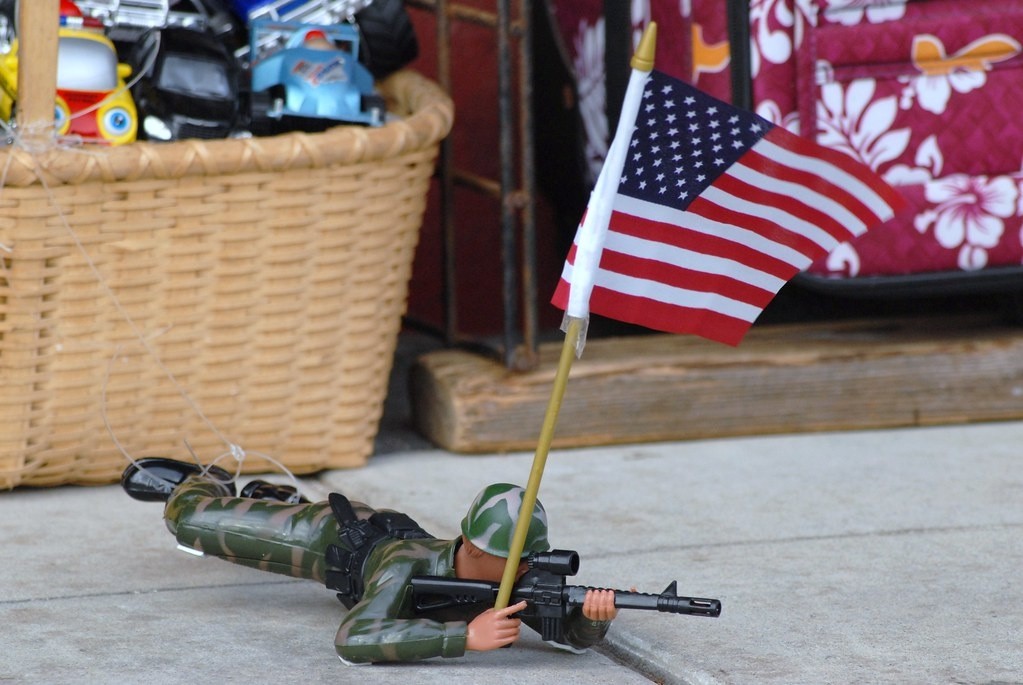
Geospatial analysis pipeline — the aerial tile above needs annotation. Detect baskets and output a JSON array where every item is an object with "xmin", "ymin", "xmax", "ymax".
[{"xmin": 0, "ymin": 72, "xmax": 452, "ymax": 490}]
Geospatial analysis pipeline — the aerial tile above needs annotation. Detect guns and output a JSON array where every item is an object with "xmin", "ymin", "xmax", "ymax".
[{"xmin": 408, "ymin": 547, "xmax": 723, "ymax": 645}]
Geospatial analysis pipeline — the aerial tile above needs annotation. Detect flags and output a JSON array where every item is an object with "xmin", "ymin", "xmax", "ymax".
[{"xmin": 549, "ymin": 71, "xmax": 909, "ymax": 348}]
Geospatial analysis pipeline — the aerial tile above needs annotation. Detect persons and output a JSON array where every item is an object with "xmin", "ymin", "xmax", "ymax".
[{"xmin": 121, "ymin": 456, "xmax": 637, "ymax": 663}]
